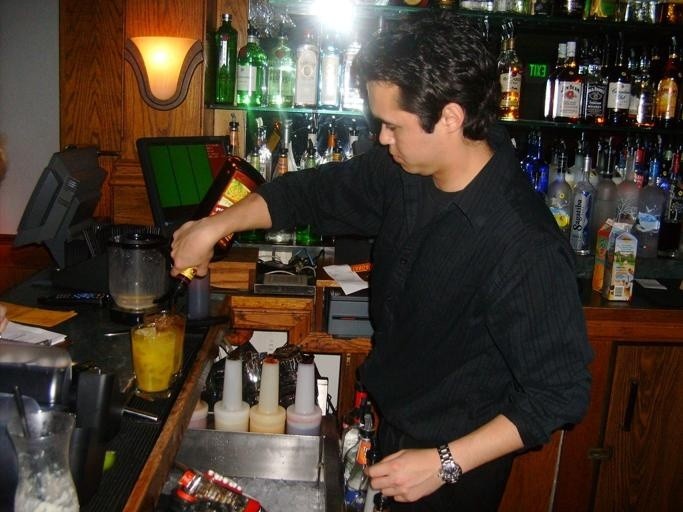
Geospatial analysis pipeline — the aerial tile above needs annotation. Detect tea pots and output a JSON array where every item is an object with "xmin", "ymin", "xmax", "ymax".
[{"xmin": 105, "ymin": 234, "xmax": 176, "ymax": 309}]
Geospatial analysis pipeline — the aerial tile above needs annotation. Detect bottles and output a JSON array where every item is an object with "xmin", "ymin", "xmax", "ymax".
[
  {"xmin": 222, "ymin": 112, "xmax": 683, "ymax": 258},
  {"xmin": 543, "ymin": 32, "xmax": 683, "ymax": 131},
  {"xmin": 187, "ymin": 348, "xmax": 322, "ymax": 437},
  {"xmin": 164, "ymin": 155, "xmax": 270, "ymax": 307},
  {"xmin": 365, "ymin": 0, "xmax": 680, "ymax": 23},
  {"xmin": 209, "ymin": 0, "xmax": 523, "ymax": 122},
  {"xmin": 340, "ymin": 397, "xmax": 389, "ymax": 511}
]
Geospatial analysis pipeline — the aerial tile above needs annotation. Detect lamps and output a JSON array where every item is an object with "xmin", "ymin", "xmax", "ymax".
[{"xmin": 127, "ymin": 35, "xmax": 204, "ymax": 112}]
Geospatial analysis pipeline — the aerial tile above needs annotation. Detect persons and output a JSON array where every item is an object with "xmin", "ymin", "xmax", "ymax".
[{"xmin": 169, "ymin": 17, "xmax": 596, "ymax": 511}]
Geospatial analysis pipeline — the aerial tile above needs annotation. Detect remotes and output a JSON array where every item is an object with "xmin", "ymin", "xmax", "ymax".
[{"xmin": 37, "ymin": 292, "xmax": 112, "ymax": 308}]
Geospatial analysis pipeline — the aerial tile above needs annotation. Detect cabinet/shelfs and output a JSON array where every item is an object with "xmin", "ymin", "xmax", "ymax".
[
  {"xmin": 541, "ymin": 2, "xmax": 679, "ymax": 266},
  {"xmin": 501, "ymin": 269, "xmax": 682, "ymax": 510},
  {"xmin": 216, "ymin": 0, "xmax": 562, "ymax": 318}
]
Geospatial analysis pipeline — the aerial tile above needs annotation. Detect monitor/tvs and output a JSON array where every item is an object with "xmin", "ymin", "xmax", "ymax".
[
  {"xmin": 137, "ymin": 135, "xmax": 233, "ymax": 231},
  {"xmin": 14, "ymin": 142, "xmax": 119, "ymax": 292}
]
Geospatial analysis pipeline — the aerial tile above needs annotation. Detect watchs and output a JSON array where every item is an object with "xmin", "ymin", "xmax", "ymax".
[{"xmin": 436, "ymin": 443, "xmax": 463, "ymax": 486}]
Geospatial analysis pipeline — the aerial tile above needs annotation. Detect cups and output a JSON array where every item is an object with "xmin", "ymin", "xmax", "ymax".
[
  {"xmin": 131, "ymin": 323, "xmax": 179, "ymax": 402},
  {"xmin": 141, "ymin": 310, "xmax": 187, "ymax": 381},
  {"xmin": 8, "ymin": 409, "xmax": 81, "ymax": 511}
]
